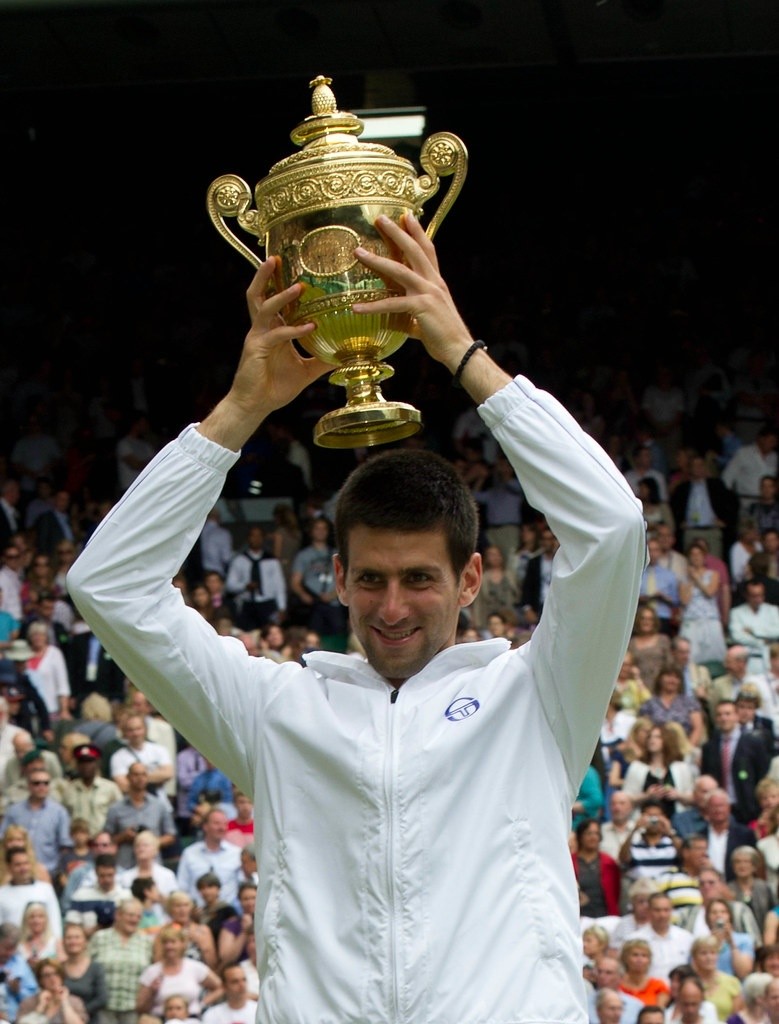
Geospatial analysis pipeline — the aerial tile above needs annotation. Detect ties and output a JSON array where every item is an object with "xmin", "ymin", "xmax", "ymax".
[{"xmin": 722, "ymin": 737, "xmax": 732, "ymax": 791}]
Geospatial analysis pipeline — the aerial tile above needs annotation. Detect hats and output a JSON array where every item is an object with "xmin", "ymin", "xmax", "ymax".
[
  {"xmin": 3, "ymin": 640, "xmax": 36, "ymax": 661},
  {"xmin": 0, "ymin": 686, "xmax": 24, "ymax": 701},
  {"xmin": 73, "ymin": 744, "xmax": 102, "ymax": 762}
]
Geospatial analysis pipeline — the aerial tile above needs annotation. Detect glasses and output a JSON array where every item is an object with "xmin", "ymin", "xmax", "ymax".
[{"xmin": 30, "ymin": 781, "xmax": 49, "ymax": 785}]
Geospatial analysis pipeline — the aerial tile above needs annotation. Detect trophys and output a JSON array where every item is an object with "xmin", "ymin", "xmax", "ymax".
[{"xmin": 207, "ymin": 74, "xmax": 470, "ymax": 449}]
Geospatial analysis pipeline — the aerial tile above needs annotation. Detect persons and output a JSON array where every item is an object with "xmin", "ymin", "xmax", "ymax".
[
  {"xmin": 65, "ymin": 212, "xmax": 651, "ymax": 1022},
  {"xmin": 178, "ymin": 808, "xmax": 246, "ymax": 904},
  {"xmin": 0, "ymin": 292, "xmax": 779, "ymax": 1024},
  {"xmin": 572, "ymin": 820, "xmax": 622, "ymax": 917}
]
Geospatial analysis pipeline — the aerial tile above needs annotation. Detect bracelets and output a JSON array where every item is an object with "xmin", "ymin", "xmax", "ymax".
[{"xmin": 453, "ymin": 340, "xmax": 486, "ymax": 384}]
